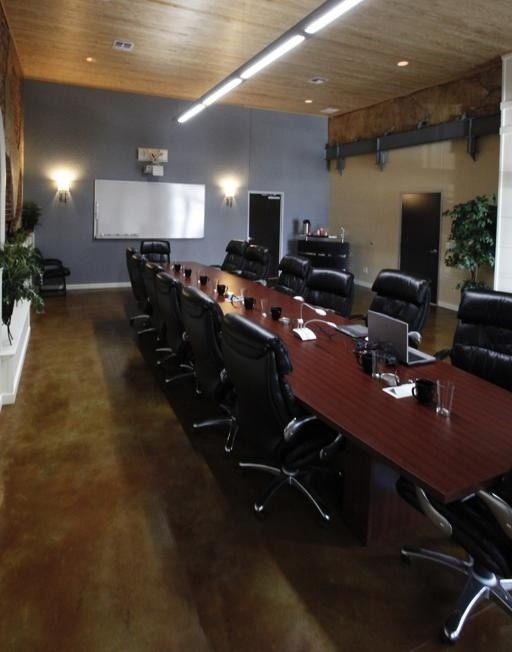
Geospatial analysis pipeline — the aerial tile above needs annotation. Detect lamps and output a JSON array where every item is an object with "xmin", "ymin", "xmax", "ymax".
[
  {"xmin": 238, "ymin": 33, "xmax": 309, "ymax": 82},
  {"xmin": 175, "ymin": 103, "xmax": 203, "ymax": 126},
  {"xmin": 50, "ymin": 163, "xmax": 75, "ymax": 202},
  {"xmin": 217, "ymin": 172, "xmax": 242, "ymax": 205},
  {"xmin": 200, "ymin": 74, "xmax": 243, "ymax": 108},
  {"xmin": 303, "ymin": 0, "xmax": 361, "ymax": 39}
]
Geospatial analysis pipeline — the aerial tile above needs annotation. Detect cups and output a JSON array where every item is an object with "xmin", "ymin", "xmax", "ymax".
[
  {"xmin": 211, "ymin": 278, "xmax": 220, "ymax": 293},
  {"xmin": 242, "ymin": 296, "xmax": 257, "ymax": 311},
  {"xmin": 172, "ymin": 264, "xmax": 182, "ymax": 273},
  {"xmin": 270, "ymin": 305, "xmax": 282, "ymax": 322},
  {"xmin": 199, "ymin": 275, "xmax": 211, "ymax": 287},
  {"xmin": 259, "ymin": 296, "xmax": 270, "ymax": 320},
  {"xmin": 435, "ymin": 379, "xmax": 456, "ymax": 418},
  {"xmin": 372, "ymin": 349, "xmax": 387, "ymax": 374},
  {"xmin": 411, "ymin": 377, "xmax": 437, "ymax": 409},
  {"xmin": 218, "ymin": 284, "xmax": 229, "ymax": 296},
  {"xmin": 196, "ymin": 268, "xmax": 204, "ymax": 284},
  {"xmin": 183, "ymin": 268, "xmax": 192, "ymax": 279},
  {"xmin": 240, "ymin": 288, "xmax": 249, "ymax": 305}
]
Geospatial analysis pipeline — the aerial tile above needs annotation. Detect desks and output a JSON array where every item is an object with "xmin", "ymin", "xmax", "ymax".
[{"xmin": 149, "ymin": 261, "xmax": 512, "ymax": 561}]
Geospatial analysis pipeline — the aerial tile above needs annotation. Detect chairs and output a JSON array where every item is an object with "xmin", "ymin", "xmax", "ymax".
[
  {"xmin": 165, "ymin": 284, "xmax": 224, "ymax": 397},
  {"xmin": 138, "ymin": 261, "xmax": 165, "ymax": 346},
  {"xmin": 221, "ymin": 239, "xmax": 247, "ymax": 276},
  {"xmin": 352, "ymin": 267, "xmax": 431, "ymax": 351},
  {"xmin": 126, "ymin": 245, "xmax": 136, "ymax": 315},
  {"xmin": 430, "ymin": 288, "xmax": 511, "ymax": 391},
  {"xmin": 243, "ymin": 242, "xmax": 271, "ymax": 285},
  {"xmin": 303, "ymin": 267, "xmax": 355, "ymax": 315},
  {"xmin": 34, "ymin": 247, "xmax": 72, "ymax": 303},
  {"xmin": 399, "ymin": 473, "xmax": 512, "ymax": 642},
  {"xmin": 191, "ymin": 313, "xmax": 346, "ymax": 522},
  {"xmin": 155, "ymin": 271, "xmax": 186, "ymax": 368},
  {"xmin": 275, "ymin": 253, "xmax": 309, "ymax": 296},
  {"xmin": 130, "ymin": 254, "xmax": 148, "ymax": 326}
]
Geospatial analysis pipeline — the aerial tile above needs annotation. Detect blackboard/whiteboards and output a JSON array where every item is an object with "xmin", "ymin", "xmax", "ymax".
[{"xmin": 93, "ymin": 178, "xmax": 206, "ymax": 239}]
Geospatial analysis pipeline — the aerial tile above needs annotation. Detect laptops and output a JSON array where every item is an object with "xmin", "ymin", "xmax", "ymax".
[{"xmin": 368, "ymin": 310, "xmax": 436, "ymax": 366}]
